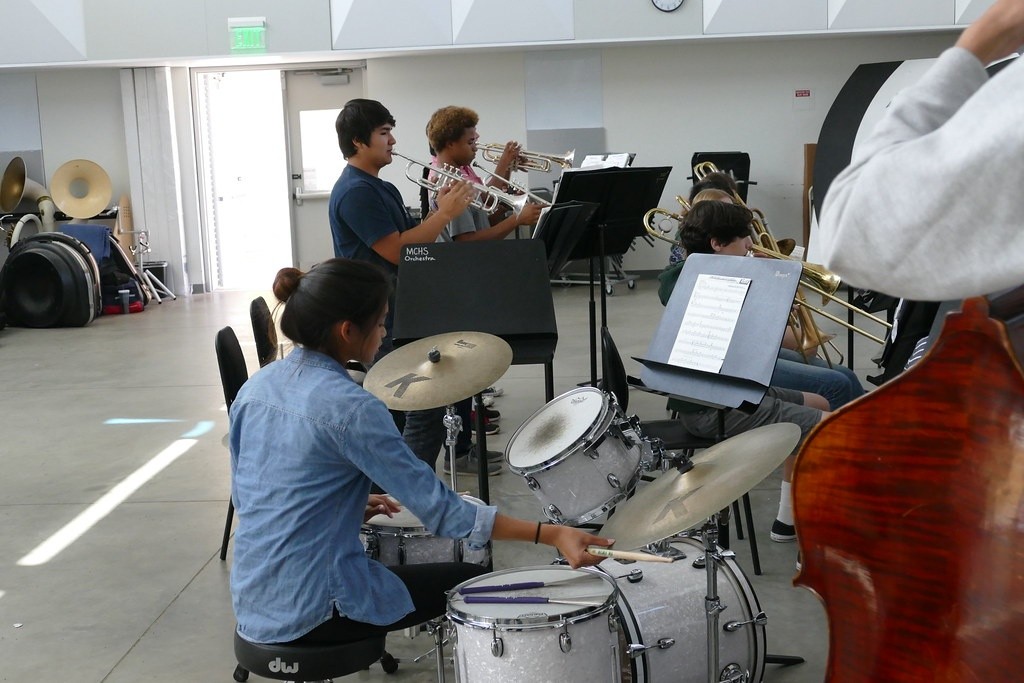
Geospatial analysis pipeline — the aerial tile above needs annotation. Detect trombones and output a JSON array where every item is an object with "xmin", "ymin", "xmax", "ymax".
[
  {"xmin": 643, "ymin": 209, "xmax": 894, "ymax": 344},
  {"xmin": 675, "ymin": 162, "xmax": 845, "ymax": 370}
]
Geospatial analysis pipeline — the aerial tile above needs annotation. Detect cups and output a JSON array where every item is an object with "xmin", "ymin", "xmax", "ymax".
[{"xmin": 118, "ymin": 290, "xmax": 130, "ymax": 314}]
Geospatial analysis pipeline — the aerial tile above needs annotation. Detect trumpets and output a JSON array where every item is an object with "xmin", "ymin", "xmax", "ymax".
[
  {"xmin": 390, "ymin": 149, "xmax": 529, "ymax": 218},
  {"xmin": 476, "ymin": 141, "xmax": 575, "ymax": 172},
  {"xmin": 472, "ymin": 161, "xmax": 551, "ymax": 206}
]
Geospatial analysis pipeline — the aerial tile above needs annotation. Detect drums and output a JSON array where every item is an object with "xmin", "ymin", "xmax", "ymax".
[
  {"xmin": 447, "ymin": 565, "xmax": 620, "ymax": 682},
  {"xmin": 596, "ymin": 532, "xmax": 766, "ymax": 683},
  {"xmin": 358, "ymin": 493, "xmax": 490, "ymax": 565},
  {"xmin": 504, "ymin": 387, "xmax": 652, "ymax": 525}
]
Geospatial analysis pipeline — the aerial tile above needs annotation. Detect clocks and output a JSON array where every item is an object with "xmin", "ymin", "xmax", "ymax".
[{"xmin": 654, "ymin": 1, "xmax": 683, "ymax": 13}]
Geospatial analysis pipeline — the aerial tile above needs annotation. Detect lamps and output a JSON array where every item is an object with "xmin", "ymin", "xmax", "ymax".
[{"xmin": 317, "ymin": 69, "xmax": 353, "ymax": 86}]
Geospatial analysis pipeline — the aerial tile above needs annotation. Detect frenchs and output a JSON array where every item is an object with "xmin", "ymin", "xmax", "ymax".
[
  {"xmin": 0, "ymin": 157, "xmax": 54, "ymax": 248},
  {"xmin": 50, "ymin": 159, "xmax": 112, "ymax": 224}
]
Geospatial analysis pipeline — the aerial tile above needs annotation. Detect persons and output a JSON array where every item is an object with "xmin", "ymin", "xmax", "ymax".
[
  {"xmin": 229, "ymin": 257, "xmax": 614, "ymax": 646},
  {"xmin": 330, "ymin": 99, "xmax": 550, "ymax": 505},
  {"xmin": 818, "ymin": 0, "xmax": 1024, "ymax": 301},
  {"xmin": 655, "ymin": 172, "xmax": 863, "ymax": 570}
]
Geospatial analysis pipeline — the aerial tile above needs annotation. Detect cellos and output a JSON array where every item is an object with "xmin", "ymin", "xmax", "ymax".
[{"xmin": 792, "ymin": 298, "xmax": 1024, "ymax": 682}]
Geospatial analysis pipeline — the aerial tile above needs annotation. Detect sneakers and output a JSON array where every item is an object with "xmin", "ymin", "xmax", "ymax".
[
  {"xmin": 475, "ymin": 405, "xmax": 500, "ymax": 422},
  {"xmin": 466, "ymin": 443, "xmax": 503, "ymax": 462},
  {"xmin": 471, "ymin": 420, "xmax": 500, "ymax": 434},
  {"xmin": 482, "ymin": 386, "xmax": 504, "ymax": 396},
  {"xmin": 471, "ymin": 396, "xmax": 494, "ymax": 407},
  {"xmin": 770, "ymin": 517, "xmax": 797, "ymax": 543},
  {"xmin": 443, "ymin": 453, "xmax": 503, "ymax": 477},
  {"xmin": 796, "ymin": 550, "xmax": 803, "ymax": 570}
]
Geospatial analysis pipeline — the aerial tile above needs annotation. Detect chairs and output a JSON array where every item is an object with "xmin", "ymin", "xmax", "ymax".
[
  {"xmin": 249, "ymin": 297, "xmax": 281, "ymax": 369},
  {"xmin": 214, "ymin": 327, "xmax": 250, "ymax": 562},
  {"xmin": 599, "ymin": 327, "xmax": 765, "ymax": 574},
  {"xmin": 799, "ymin": 143, "xmax": 894, "ymax": 373}
]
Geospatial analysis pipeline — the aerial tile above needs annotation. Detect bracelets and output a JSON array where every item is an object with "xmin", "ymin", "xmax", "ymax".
[{"xmin": 534, "ymin": 521, "xmax": 542, "ymax": 545}]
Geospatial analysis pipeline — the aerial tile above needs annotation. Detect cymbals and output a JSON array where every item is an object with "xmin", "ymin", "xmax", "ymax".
[
  {"xmin": 363, "ymin": 331, "xmax": 513, "ymax": 410},
  {"xmin": 586, "ymin": 423, "xmax": 803, "ymax": 553}
]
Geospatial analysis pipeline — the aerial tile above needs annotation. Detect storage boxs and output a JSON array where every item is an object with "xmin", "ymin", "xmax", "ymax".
[{"xmin": 135, "ymin": 262, "xmax": 167, "ymax": 298}]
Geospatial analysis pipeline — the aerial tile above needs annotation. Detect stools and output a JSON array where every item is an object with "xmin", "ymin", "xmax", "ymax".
[{"xmin": 231, "ymin": 626, "xmax": 398, "ymax": 683}]
